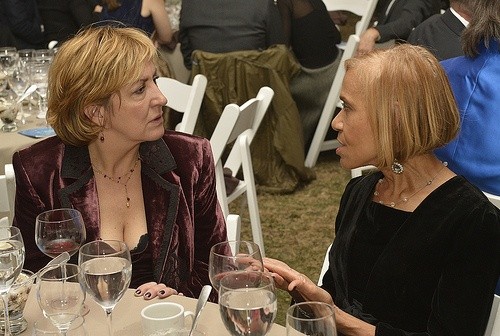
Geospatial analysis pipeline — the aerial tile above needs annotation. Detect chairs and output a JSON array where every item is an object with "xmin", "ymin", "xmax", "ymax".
[
  {"xmin": 303, "ymin": 0, "xmax": 398, "ymax": 169},
  {"xmin": 210, "ymin": 87, "xmax": 276, "ymax": 256},
  {"xmin": 155, "ymin": 70, "xmax": 208, "ymax": 137}
]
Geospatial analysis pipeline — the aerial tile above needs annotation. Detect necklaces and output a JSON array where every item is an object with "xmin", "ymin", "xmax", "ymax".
[
  {"xmin": 372, "ymin": 162, "xmax": 447, "ymax": 207},
  {"xmin": 90, "ymin": 152, "xmax": 140, "ymax": 208}
]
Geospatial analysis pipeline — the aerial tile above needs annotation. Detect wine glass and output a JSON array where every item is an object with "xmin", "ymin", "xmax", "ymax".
[
  {"xmin": 0, "ymin": 225, "xmax": 25, "ymax": 336},
  {"xmin": 77, "ymin": 239, "xmax": 132, "ymax": 336},
  {"xmin": 35, "ymin": 209, "xmax": 91, "ymax": 317},
  {"xmin": 0, "ymin": 46, "xmax": 55, "ymax": 133},
  {"xmin": 36, "ymin": 263, "xmax": 86, "ymax": 336}
]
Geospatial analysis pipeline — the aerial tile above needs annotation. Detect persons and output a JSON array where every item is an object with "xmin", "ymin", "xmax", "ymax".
[{"xmin": 1, "ymin": 0, "xmax": 500, "ymax": 336}]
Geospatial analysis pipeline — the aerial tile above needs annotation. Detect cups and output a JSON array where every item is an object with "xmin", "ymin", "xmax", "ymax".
[
  {"xmin": 154, "ymin": 328, "xmax": 207, "ymax": 336},
  {"xmin": 140, "ymin": 303, "xmax": 194, "ymax": 336},
  {"xmin": 0, "ymin": 269, "xmax": 35, "ymax": 336},
  {"xmin": 209, "ymin": 240, "xmax": 264, "ymax": 292},
  {"xmin": 217, "ymin": 271, "xmax": 278, "ymax": 336},
  {"xmin": 285, "ymin": 301, "xmax": 337, "ymax": 336},
  {"xmin": 33, "ymin": 312, "xmax": 87, "ymax": 336}
]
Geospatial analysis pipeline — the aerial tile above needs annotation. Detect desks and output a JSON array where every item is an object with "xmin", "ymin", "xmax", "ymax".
[
  {"xmin": 0, "ymin": 282, "xmax": 289, "ymax": 336},
  {"xmin": 0, "ymin": 104, "xmax": 54, "ymax": 173}
]
